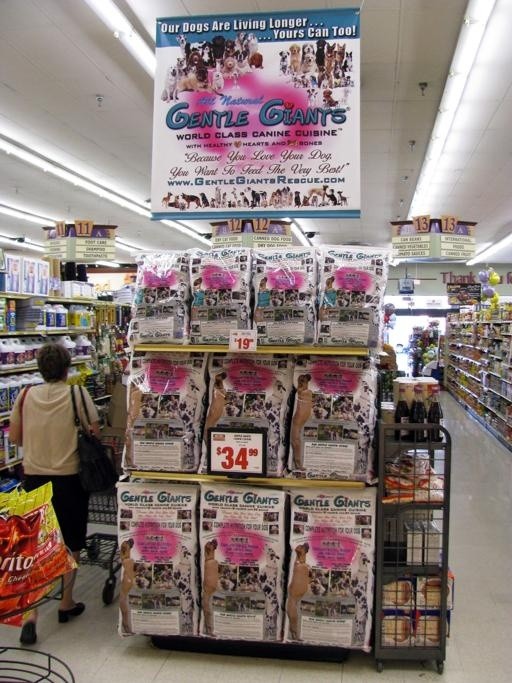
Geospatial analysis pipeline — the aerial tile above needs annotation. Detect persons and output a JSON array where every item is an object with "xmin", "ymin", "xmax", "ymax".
[{"xmin": 8, "ymin": 344, "xmax": 101, "ymax": 644}]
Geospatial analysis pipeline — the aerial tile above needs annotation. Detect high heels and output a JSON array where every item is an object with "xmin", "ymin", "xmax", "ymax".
[
  {"xmin": 58, "ymin": 602, "xmax": 85, "ymax": 623},
  {"xmin": 20, "ymin": 621, "xmax": 37, "ymax": 644}
]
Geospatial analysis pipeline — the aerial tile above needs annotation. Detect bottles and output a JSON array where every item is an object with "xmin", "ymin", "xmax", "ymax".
[
  {"xmin": 394, "ymin": 388, "xmax": 411, "ymax": 442},
  {"xmin": 409, "ymin": 385, "xmax": 426, "ymax": 442},
  {"xmin": 427, "ymin": 387, "xmax": 444, "ymax": 442}
]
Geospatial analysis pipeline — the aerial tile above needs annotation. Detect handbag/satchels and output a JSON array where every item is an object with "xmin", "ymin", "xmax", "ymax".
[{"xmin": 71, "ymin": 385, "xmax": 119, "ymax": 493}]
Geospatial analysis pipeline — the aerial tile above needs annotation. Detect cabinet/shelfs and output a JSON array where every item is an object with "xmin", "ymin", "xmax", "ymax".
[
  {"xmin": 1, "ymin": 295, "xmax": 128, "ymax": 468},
  {"xmin": 127, "ymin": 346, "xmax": 450, "ymax": 674},
  {"xmin": 443, "ymin": 315, "xmax": 511, "ymax": 449}
]
